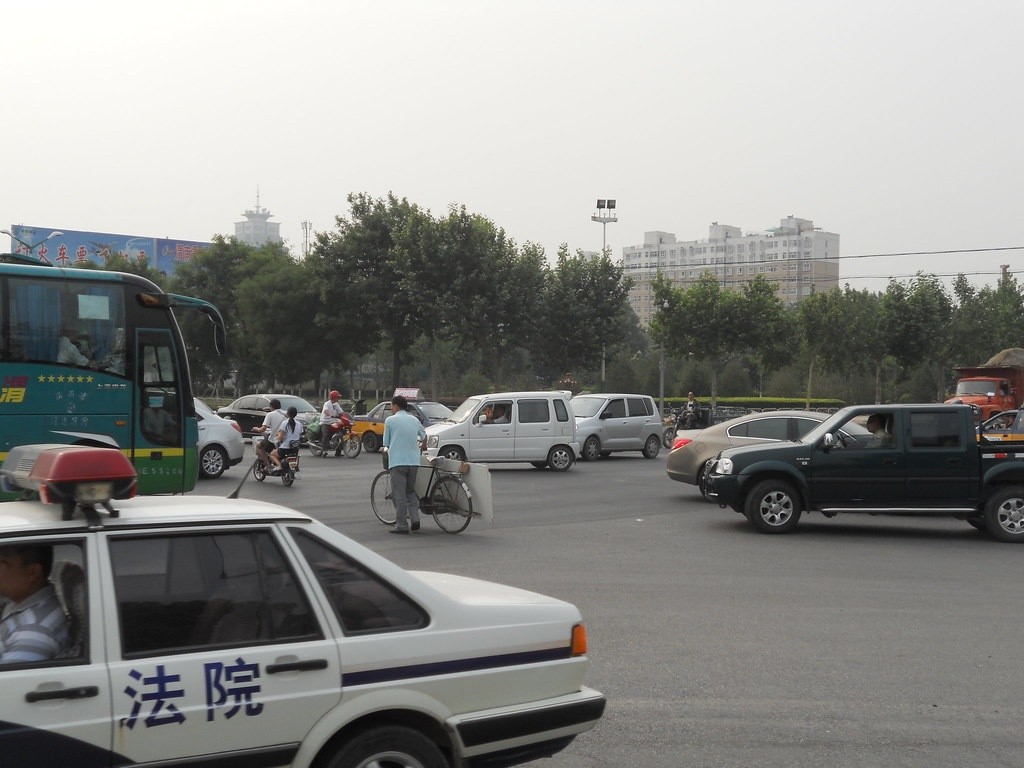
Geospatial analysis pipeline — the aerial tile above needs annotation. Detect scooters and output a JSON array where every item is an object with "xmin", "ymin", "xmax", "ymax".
[{"xmin": 250, "ymin": 429, "xmax": 302, "ymax": 487}]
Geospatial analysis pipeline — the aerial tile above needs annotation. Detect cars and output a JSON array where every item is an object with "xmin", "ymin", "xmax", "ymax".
[
  {"xmin": 975, "ymin": 403, "xmax": 1024, "ymax": 443},
  {"xmin": 665, "ymin": 409, "xmax": 873, "ymax": 504},
  {"xmin": 150, "ymin": 391, "xmax": 245, "ymax": 480},
  {"xmin": 350, "ymin": 387, "xmax": 454, "ymax": 454},
  {"xmin": 0, "ymin": 442, "xmax": 608, "ymax": 768},
  {"xmin": 216, "ymin": 393, "xmax": 320, "ymax": 444}
]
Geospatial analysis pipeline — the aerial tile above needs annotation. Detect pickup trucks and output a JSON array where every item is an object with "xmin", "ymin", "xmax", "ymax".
[{"xmin": 706, "ymin": 399, "xmax": 1024, "ymax": 544}]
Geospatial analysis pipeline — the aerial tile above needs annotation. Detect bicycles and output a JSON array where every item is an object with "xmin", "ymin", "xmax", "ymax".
[{"xmin": 370, "ymin": 444, "xmax": 482, "ymax": 535}]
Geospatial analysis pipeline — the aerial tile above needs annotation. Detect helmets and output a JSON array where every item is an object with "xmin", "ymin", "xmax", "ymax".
[{"xmin": 330, "ymin": 391, "xmax": 341, "ymax": 399}]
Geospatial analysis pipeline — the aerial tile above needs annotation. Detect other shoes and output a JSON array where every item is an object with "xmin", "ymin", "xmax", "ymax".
[
  {"xmin": 411, "ymin": 522, "xmax": 420, "ymax": 531},
  {"xmin": 260, "ymin": 467, "xmax": 269, "ymax": 472},
  {"xmin": 273, "ymin": 465, "xmax": 283, "ymax": 471},
  {"xmin": 390, "ymin": 527, "xmax": 409, "ymax": 534},
  {"xmin": 321, "ymin": 453, "xmax": 327, "ymax": 457},
  {"xmin": 335, "ymin": 452, "xmax": 345, "ymax": 456}
]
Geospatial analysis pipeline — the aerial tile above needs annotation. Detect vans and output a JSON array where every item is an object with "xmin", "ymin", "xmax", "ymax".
[
  {"xmin": 559, "ymin": 390, "xmax": 665, "ymax": 460},
  {"xmin": 417, "ymin": 389, "xmax": 580, "ymax": 473}
]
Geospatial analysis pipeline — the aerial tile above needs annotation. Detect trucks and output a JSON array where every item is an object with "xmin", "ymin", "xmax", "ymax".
[{"xmin": 942, "ymin": 364, "xmax": 1024, "ymax": 427}]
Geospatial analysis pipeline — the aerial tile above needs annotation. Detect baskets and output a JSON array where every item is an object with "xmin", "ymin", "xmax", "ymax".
[{"xmin": 381, "ymin": 451, "xmax": 388, "ymax": 470}]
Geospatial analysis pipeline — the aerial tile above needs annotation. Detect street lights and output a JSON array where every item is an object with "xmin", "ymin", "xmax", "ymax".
[{"xmin": 591, "ymin": 199, "xmax": 619, "ymax": 379}]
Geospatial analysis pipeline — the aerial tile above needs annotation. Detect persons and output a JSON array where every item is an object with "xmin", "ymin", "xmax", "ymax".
[
  {"xmin": 253, "ymin": 399, "xmax": 288, "ymax": 472},
  {"xmin": 0, "ymin": 543, "xmax": 70, "ymax": 663},
  {"xmin": 57, "ymin": 321, "xmax": 96, "ymax": 366},
  {"xmin": 493, "ymin": 405, "xmax": 508, "ymax": 424},
  {"xmin": 270, "ymin": 407, "xmax": 304, "ymax": 471},
  {"xmin": 1001, "ymin": 415, "xmax": 1014, "ymax": 430},
  {"xmin": 865, "ymin": 415, "xmax": 890, "ymax": 448},
  {"xmin": 319, "ymin": 391, "xmax": 346, "ymax": 457},
  {"xmin": 383, "ymin": 396, "xmax": 428, "ymax": 534},
  {"xmin": 681, "ymin": 391, "xmax": 702, "ymax": 429}
]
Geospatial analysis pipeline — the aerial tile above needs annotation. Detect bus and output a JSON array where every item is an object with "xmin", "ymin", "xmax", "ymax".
[{"xmin": 0, "ymin": 252, "xmax": 228, "ymax": 501}]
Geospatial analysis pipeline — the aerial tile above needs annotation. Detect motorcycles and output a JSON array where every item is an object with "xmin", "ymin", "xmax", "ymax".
[
  {"xmin": 304, "ymin": 411, "xmax": 362, "ymax": 459},
  {"xmin": 662, "ymin": 405, "xmax": 748, "ymax": 449}
]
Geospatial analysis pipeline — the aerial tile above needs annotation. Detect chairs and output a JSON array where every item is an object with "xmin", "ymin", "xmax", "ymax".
[
  {"xmin": 887, "ymin": 418, "xmax": 893, "ymax": 434},
  {"xmin": 60, "ymin": 560, "xmax": 86, "ymax": 657}
]
